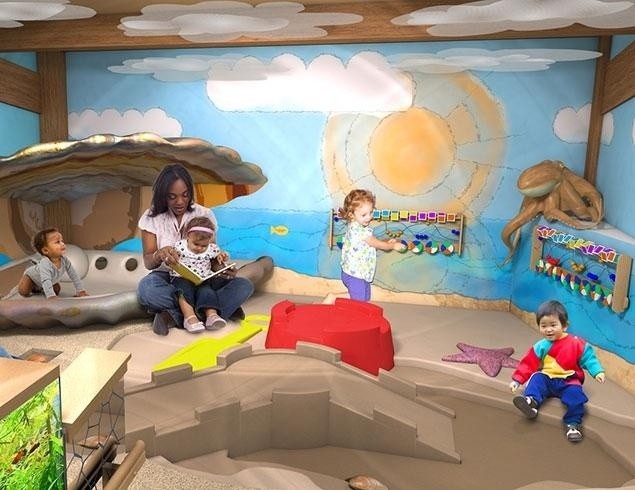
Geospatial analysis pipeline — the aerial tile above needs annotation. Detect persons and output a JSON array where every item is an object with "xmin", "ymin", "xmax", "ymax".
[
  {"xmin": 137, "ymin": 164, "xmax": 255, "ymax": 335},
  {"xmin": 167, "ymin": 218, "xmax": 228, "ymax": 332},
  {"xmin": 320, "ymin": 189, "xmax": 396, "ymax": 306},
  {"xmin": 18, "ymin": 229, "xmax": 89, "ymax": 301},
  {"xmin": 510, "ymin": 300, "xmax": 606, "ymax": 443}
]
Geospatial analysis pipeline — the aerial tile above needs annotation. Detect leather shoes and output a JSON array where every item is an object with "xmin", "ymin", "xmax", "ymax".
[
  {"xmin": 153, "ymin": 310, "xmax": 174, "ymax": 336},
  {"xmin": 230, "ymin": 308, "xmax": 245, "ymax": 320},
  {"xmin": 206, "ymin": 314, "xmax": 226, "ymax": 330},
  {"xmin": 182, "ymin": 316, "xmax": 205, "ymax": 332}
]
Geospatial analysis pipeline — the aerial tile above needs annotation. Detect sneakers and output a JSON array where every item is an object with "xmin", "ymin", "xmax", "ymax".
[
  {"xmin": 512, "ymin": 395, "xmax": 538, "ymax": 419},
  {"xmin": 565, "ymin": 423, "xmax": 583, "ymax": 442}
]
